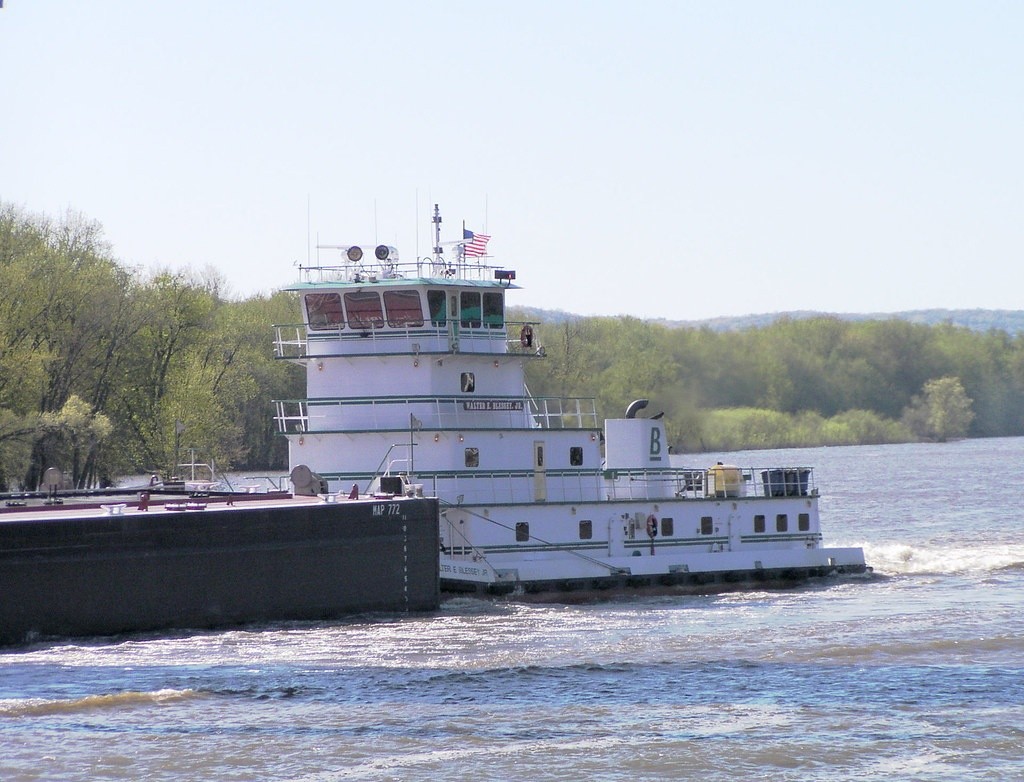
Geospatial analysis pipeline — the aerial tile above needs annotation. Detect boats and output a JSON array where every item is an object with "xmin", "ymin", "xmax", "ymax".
[{"xmin": 271, "ymin": 185, "xmax": 875, "ymax": 594}]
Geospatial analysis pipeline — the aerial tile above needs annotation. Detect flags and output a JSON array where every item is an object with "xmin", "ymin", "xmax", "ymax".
[{"xmin": 463, "ymin": 230, "xmax": 491, "ymax": 258}]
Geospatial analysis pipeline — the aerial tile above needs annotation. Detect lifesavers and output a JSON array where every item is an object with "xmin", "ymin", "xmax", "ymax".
[
  {"xmin": 521, "ymin": 327, "xmax": 533, "ymax": 345},
  {"xmin": 647, "ymin": 515, "xmax": 658, "ymax": 536}
]
